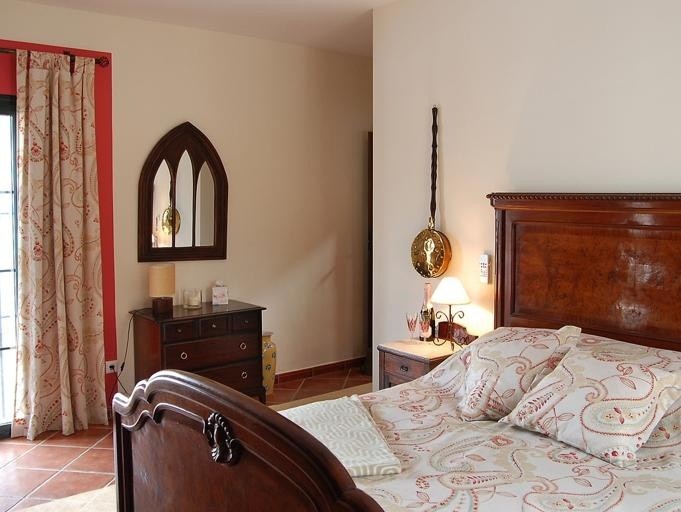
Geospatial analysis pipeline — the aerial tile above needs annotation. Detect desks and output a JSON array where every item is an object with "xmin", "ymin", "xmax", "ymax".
[{"xmin": 128, "ymin": 300, "xmax": 267, "ymax": 406}]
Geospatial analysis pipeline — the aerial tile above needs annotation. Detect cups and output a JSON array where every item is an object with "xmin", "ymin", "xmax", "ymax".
[{"xmin": 183, "ymin": 289, "xmax": 202, "ymax": 309}]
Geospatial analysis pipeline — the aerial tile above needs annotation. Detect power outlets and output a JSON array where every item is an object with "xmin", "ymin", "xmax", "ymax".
[{"xmin": 105, "ymin": 360, "xmax": 119, "ymax": 374}]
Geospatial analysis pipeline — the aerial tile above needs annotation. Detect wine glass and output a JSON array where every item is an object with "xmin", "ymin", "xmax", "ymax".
[{"xmin": 406, "ymin": 312, "xmax": 418, "ymax": 344}]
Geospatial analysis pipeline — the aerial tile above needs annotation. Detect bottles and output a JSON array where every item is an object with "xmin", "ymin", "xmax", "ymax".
[{"xmin": 418, "ymin": 282, "xmax": 435, "ymax": 342}]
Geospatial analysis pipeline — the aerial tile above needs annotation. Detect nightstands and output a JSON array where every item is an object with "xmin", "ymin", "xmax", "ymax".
[{"xmin": 377, "ymin": 337, "xmax": 470, "ymax": 391}]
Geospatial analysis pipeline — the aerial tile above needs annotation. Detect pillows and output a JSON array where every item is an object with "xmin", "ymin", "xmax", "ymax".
[
  {"xmin": 497, "ymin": 345, "xmax": 681, "ymax": 472},
  {"xmin": 420, "ymin": 325, "xmax": 582, "ymax": 421}
]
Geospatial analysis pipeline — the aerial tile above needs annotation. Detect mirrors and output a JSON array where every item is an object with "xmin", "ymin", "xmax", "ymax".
[{"xmin": 137, "ymin": 121, "xmax": 228, "ymax": 262}]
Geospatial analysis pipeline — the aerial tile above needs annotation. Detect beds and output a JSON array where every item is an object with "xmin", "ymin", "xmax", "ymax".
[{"xmin": 111, "ymin": 192, "xmax": 681, "ymax": 512}]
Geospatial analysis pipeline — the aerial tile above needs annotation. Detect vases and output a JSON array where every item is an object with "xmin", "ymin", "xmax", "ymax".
[{"xmin": 262, "ymin": 332, "xmax": 276, "ymax": 396}]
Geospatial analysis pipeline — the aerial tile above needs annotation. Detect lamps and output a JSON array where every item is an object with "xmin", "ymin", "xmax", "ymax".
[
  {"xmin": 147, "ymin": 261, "xmax": 175, "ymax": 319},
  {"xmin": 430, "ymin": 276, "xmax": 472, "ymax": 351}
]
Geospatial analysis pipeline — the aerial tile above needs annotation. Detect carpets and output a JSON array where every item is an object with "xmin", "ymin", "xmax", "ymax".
[
  {"xmin": 267, "ymin": 381, "xmax": 372, "ymax": 412},
  {"xmin": 18, "ymin": 484, "xmax": 118, "ymax": 512}
]
